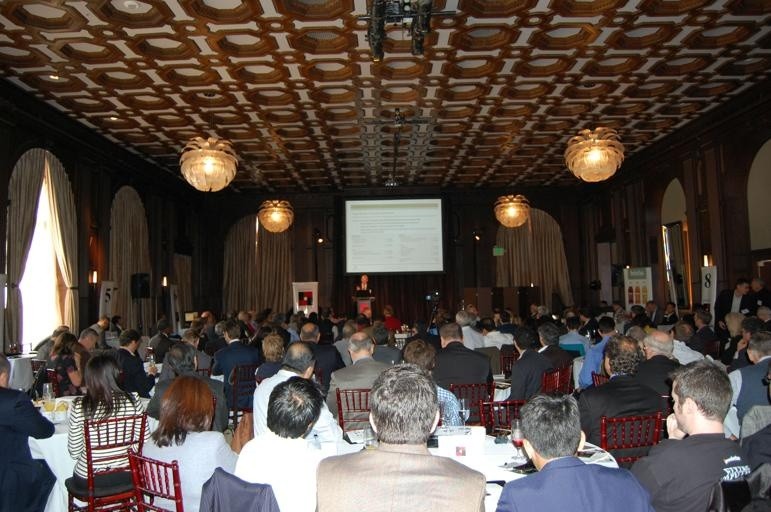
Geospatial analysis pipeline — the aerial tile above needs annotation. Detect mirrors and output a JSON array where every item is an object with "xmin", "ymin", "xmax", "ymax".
[{"xmin": 661, "ymin": 221, "xmax": 691, "ymax": 310}]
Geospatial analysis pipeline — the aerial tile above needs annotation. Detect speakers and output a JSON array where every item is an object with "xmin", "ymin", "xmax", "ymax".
[{"xmin": 130, "ymin": 273, "xmax": 151, "ymax": 299}]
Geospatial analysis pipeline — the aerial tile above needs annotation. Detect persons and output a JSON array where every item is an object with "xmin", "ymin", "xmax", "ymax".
[
  {"xmin": 631, "ymin": 361, "xmax": 754, "ymax": 511},
  {"xmin": 142, "ymin": 373, "xmax": 238, "ymax": 511},
  {"xmin": 1, "ymin": 353, "xmax": 57, "ymax": 512},
  {"xmin": 577, "ymin": 333, "xmax": 670, "ymax": 469},
  {"xmin": 496, "ymin": 393, "xmax": 657, "ymax": 512},
  {"xmin": 68, "ymin": 350, "xmax": 153, "ymax": 487},
  {"xmin": 33, "ymin": 274, "xmax": 770, "ymax": 440},
  {"xmin": 316, "ymin": 364, "xmax": 487, "ymax": 512},
  {"xmin": 743, "ymin": 369, "xmax": 771, "ymax": 473},
  {"xmin": 234, "ymin": 377, "xmax": 360, "ymax": 512}
]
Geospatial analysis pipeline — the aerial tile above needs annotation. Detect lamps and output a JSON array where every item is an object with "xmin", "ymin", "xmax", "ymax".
[
  {"xmin": 257, "ymin": 200, "xmax": 294, "ymax": 233},
  {"xmin": 179, "ymin": 136, "xmax": 239, "ymax": 192},
  {"xmin": 494, "ymin": 195, "xmax": 531, "ymax": 228},
  {"xmin": 565, "ymin": 127, "xmax": 625, "ymax": 182}
]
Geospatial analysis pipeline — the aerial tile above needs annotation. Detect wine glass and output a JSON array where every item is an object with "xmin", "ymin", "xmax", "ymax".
[
  {"xmin": 441, "ymin": 403, "xmax": 454, "ymax": 434},
  {"xmin": 149, "ymin": 354, "xmax": 155, "ymax": 366},
  {"xmin": 362, "ymin": 424, "xmax": 378, "ymax": 451},
  {"xmin": 511, "ymin": 419, "xmax": 523, "ymax": 460},
  {"xmin": 458, "ymin": 398, "xmax": 470, "ymax": 432},
  {"xmin": 43, "ymin": 384, "xmax": 58, "ymax": 423}
]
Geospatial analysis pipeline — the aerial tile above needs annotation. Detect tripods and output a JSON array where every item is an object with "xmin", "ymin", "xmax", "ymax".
[{"xmin": 424, "ymin": 305, "xmax": 442, "ymax": 334}]
